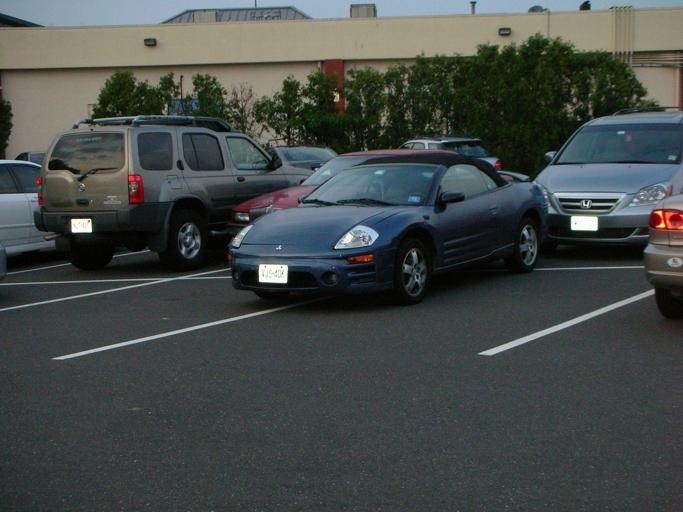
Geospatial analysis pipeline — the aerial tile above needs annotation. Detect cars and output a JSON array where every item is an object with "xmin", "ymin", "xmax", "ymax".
[
  {"xmin": 262, "ymin": 144, "xmax": 340, "ymax": 172},
  {"xmin": 639, "ymin": 190, "xmax": 682, "ymax": 324},
  {"xmin": 225, "ymin": 149, "xmax": 531, "ymax": 236},
  {"xmin": 397, "ymin": 134, "xmax": 504, "ymax": 171},
  {"xmin": 529, "ymin": 104, "xmax": 682, "ymax": 255},
  {"xmin": 0, "ymin": 158, "xmax": 62, "ymax": 282},
  {"xmin": 11, "ymin": 151, "xmax": 48, "ymax": 165}
]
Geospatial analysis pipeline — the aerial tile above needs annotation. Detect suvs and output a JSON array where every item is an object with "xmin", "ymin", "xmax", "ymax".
[{"xmin": 30, "ymin": 113, "xmax": 316, "ymax": 273}]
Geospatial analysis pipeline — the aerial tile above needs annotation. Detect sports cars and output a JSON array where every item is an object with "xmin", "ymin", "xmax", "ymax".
[{"xmin": 225, "ymin": 149, "xmax": 551, "ymax": 307}]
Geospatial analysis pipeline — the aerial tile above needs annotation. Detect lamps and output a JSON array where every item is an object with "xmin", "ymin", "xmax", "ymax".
[
  {"xmin": 498, "ymin": 26, "xmax": 510, "ymax": 36},
  {"xmin": 143, "ymin": 38, "xmax": 156, "ymax": 47}
]
